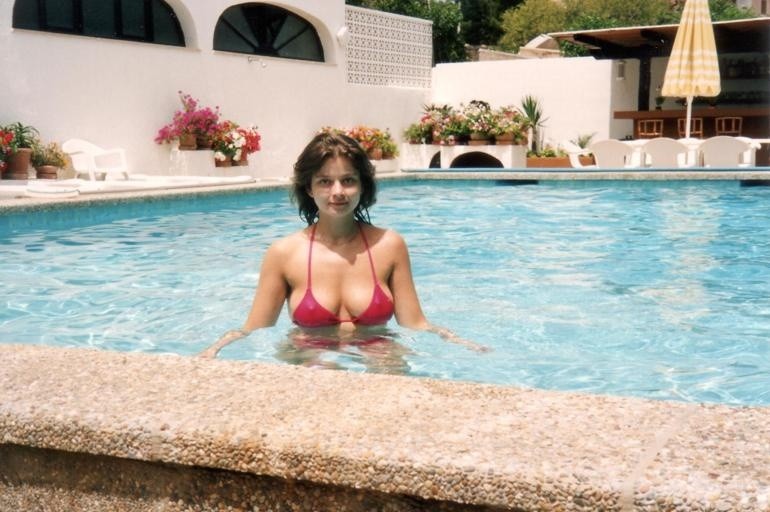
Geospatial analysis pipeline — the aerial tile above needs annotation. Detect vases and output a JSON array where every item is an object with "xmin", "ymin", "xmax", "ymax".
[
  {"xmin": 36, "ymin": 165, "xmax": 62, "ymax": 181},
  {"xmin": 5, "ymin": 147, "xmax": 37, "ymax": 182}
]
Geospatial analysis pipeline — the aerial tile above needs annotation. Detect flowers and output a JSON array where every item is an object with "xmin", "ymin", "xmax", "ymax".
[
  {"xmin": 0, "ymin": 122, "xmax": 40, "ymax": 162},
  {"xmin": 323, "ymin": 123, "xmax": 400, "ymax": 159},
  {"xmin": 152, "ymin": 90, "xmax": 269, "ymax": 167},
  {"xmin": 32, "ymin": 140, "xmax": 69, "ymax": 169},
  {"xmin": 403, "ymin": 91, "xmax": 534, "ymax": 147}
]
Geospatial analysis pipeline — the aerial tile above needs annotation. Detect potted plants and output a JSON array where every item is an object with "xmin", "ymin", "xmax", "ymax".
[{"xmin": 571, "ymin": 132, "xmax": 594, "ymax": 166}]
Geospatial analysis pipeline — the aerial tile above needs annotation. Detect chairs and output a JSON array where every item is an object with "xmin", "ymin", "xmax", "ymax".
[
  {"xmin": 61, "ymin": 136, "xmax": 133, "ymax": 186},
  {"xmin": 587, "ymin": 115, "xmax": 764, "ymax": 177}
]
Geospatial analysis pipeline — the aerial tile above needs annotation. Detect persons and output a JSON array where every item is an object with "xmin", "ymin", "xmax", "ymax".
[{"xmin": 193, "ymin": 130, "xmax": 495, "ymax": 358}]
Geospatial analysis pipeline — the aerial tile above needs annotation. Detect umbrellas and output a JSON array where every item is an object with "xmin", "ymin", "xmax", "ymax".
[{"xmin": 659, "ymin": 0, "xmax": 721, "ymax": 137}]
